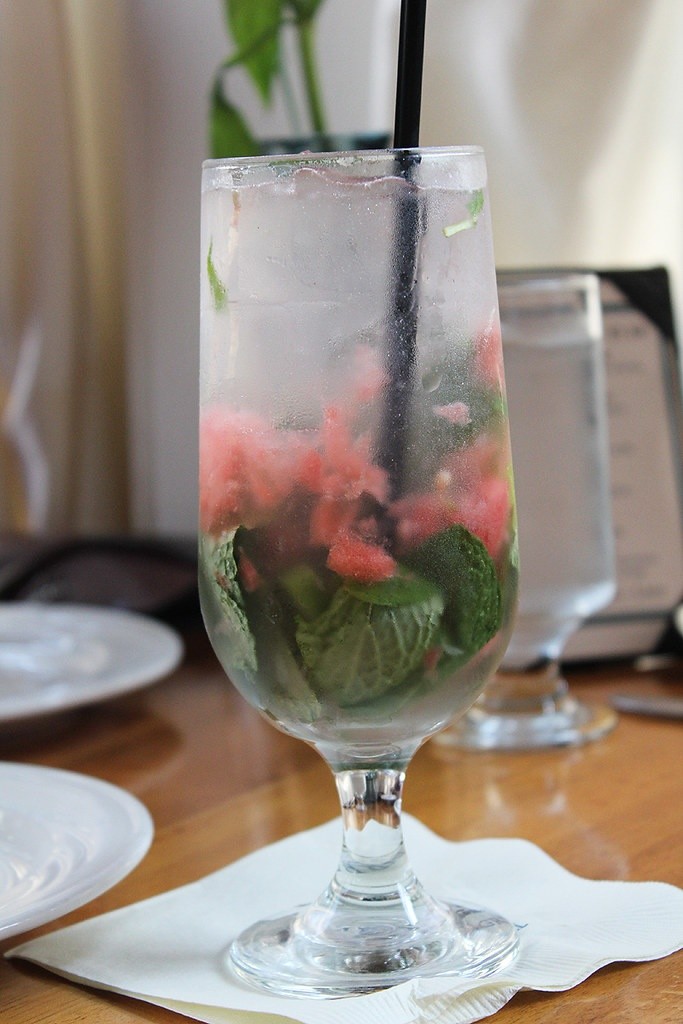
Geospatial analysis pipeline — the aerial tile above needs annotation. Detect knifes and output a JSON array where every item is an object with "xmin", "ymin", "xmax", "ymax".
[{"xmin": 606, "ymin": 694, "xmax": 683, "ymax": 719}]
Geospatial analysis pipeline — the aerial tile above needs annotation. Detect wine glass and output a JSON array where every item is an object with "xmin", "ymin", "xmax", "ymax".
[
  {"xmin": 194, "ymin": 147, "xmax": 524, "ymax": 997},
  {"xmin": 430, "ymin": 274, "xmax": 619, "ymax": 752}
]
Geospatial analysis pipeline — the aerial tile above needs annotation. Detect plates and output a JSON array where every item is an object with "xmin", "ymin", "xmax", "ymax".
[
  {"xmin": 0, "ymin": 601, "xmax": 184, "ymax": 718},
  {"xmin": 0, "ymin": 759, "xmax": 155, "ymax": 938}
]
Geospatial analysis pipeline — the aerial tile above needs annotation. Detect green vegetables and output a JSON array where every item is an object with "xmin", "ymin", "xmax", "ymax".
[{"xmin": 193, "ymin": 322, "xmax": 524, "ymax": 716}]
